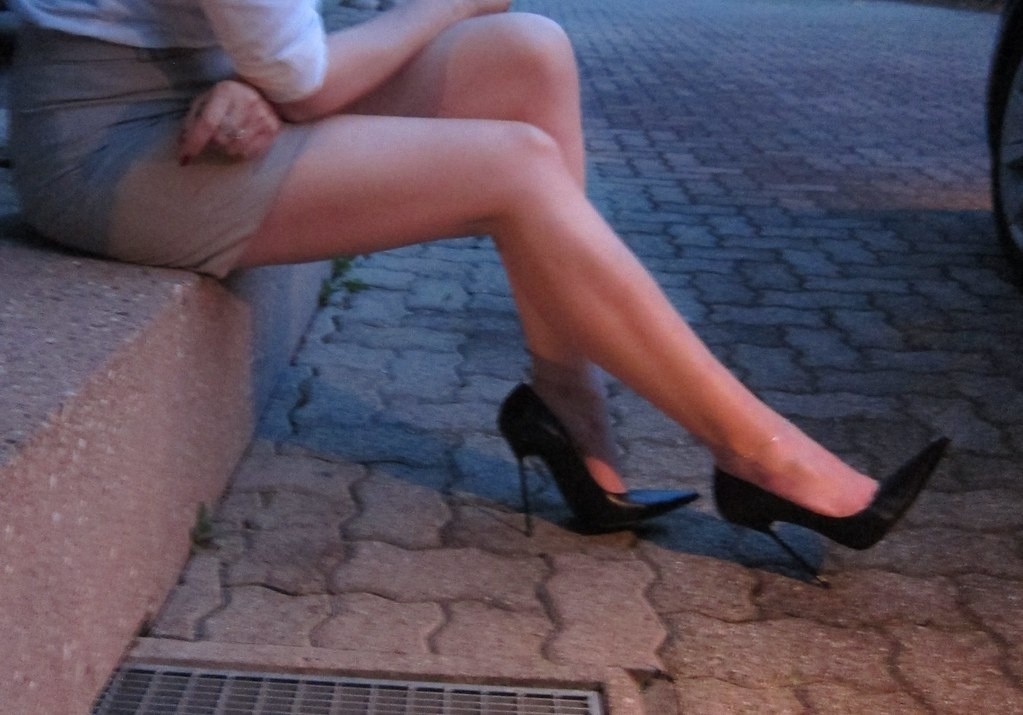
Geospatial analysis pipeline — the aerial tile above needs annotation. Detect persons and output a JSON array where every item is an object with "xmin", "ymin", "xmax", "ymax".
[{"xmin": 0, "ymin": 0, "xmax": 954, "ymax": 589}]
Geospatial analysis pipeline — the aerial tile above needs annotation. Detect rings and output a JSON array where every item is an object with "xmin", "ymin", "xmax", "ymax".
[{"xmin": 234, "ymin": 126, "xmax": 249, "ymax": 139}]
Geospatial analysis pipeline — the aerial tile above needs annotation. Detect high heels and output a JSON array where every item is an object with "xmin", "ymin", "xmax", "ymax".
[
  {"xmin": 713, "ymin": 436, "xmax": 950, "ymax": 574},
  {"xmin": 500, "ymin": 382, "xmax": 703, "ymax": 536}
]
